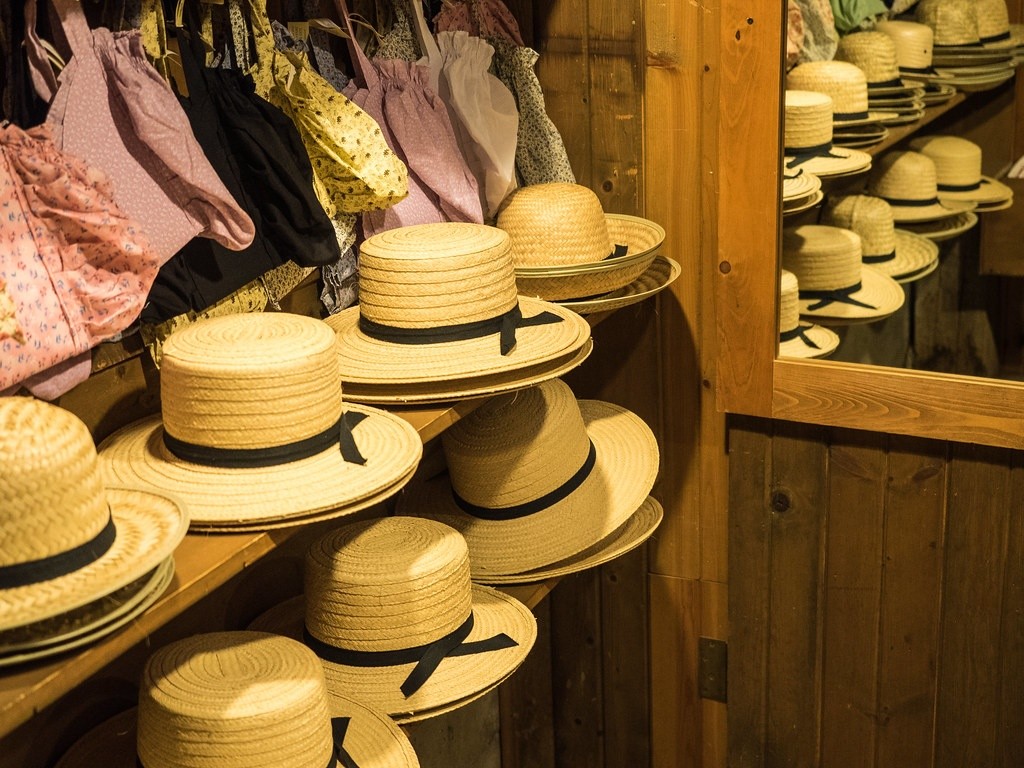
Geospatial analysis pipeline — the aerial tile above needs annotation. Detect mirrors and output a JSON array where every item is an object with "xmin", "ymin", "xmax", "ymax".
[{"xmin": 713, "ymin": 0, "xmax": 1024, "ymax": 450}]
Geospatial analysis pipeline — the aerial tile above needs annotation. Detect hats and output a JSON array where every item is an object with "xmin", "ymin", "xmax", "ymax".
[
  {"xmin": 1, "ymin": 184, "xmax": 684, "ymax": 768},
  {"xmin": 777, "ymin": 0, "xmax": 1024, "ymax": 360}
]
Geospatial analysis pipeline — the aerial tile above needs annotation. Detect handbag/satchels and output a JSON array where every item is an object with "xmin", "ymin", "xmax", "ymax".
[{"xmin": 0, "ymin": 0, "xmax": 579, "ymax": 406}]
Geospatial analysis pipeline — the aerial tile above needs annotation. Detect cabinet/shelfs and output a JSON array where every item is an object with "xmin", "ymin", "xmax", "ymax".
[
  {"xmin": 0, "ymin": 307, "xmax": 638, "ymax": 768},
  {"xmin": 828, "ymin": 76, "xmax": 1015, "ymax": 326}
]
